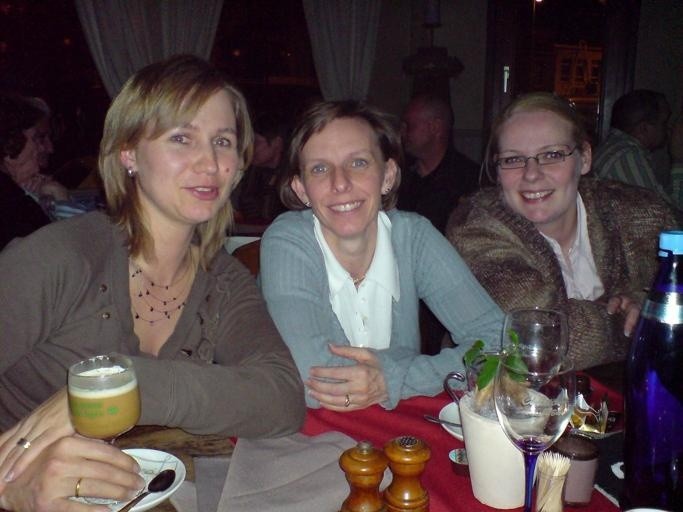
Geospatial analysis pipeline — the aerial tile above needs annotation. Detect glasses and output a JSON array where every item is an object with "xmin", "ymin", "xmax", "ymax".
[{"xmin": 496, "ymin": 145, "xmax": 576, "ymax": 169}]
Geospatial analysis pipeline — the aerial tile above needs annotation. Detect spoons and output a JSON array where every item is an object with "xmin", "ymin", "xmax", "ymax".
[{"xmin": 117, "ymin": 470, "xmax": 175, "ymax": 512}]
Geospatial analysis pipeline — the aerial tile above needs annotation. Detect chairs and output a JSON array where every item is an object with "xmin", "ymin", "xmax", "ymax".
[{"xmin": 222, "ymin": 236, "xmax": 261, "ymax": 280}]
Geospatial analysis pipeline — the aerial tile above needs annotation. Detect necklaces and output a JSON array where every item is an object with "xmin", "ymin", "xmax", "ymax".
[
  {"xmin": 351, "ymin": 274, "xmax": 367, "ymax": 286},
  {"xmin": 126, "ymin": 254, "xmax": 200, "ymax": 324}
]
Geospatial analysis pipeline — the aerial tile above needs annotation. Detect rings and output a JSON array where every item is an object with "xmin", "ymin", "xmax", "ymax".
[
  {"xmin": 74, "ymin": 477, "xmax": 85, "ymax": 500},
  {"xmin": 17, "ymin": 436, "xmax": 32, "ymax": 449},
  {"xmin": 344, "ymin": 392, "xmax": 351, "ymax": 408}
]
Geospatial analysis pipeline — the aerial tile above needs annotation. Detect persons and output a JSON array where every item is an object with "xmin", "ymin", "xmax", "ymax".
[
  {"xmin": 229, "ymin": 111, "xmax": 294, "ymax": 225},
  {"xmin": 0, "ymin": 54, "xmax": 309, "ymax": 512},
  {"xmin": 0, "ymin": 82, "xmax": 112, "ymax": 251},
  {"xmin": 393, "ymin": 94, "xmax": 468, "ymax": 358},
  {"xmin": 592, "ymin": 89, "xmax": 683, "ymax": 213},
  {"xmin": 441, "ymin": 90, "xmax": 682, "ymax": 374},
  {"xmin": 256, "ymin": 98, "xmax": 512, "ymax": 416}
]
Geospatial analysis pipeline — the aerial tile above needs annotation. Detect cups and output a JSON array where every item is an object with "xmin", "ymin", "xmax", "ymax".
[
  {"xmin": 463, "ymin": 349, "xmax": 501, "ymax": 393},
  {"xmin": 460, "ymin": 387, "xmax": 551, "ymax": 509},
  {"xmin": 550, "ymin": 432, "xmax": 597, "ymax": 506},
  {"xmin": 449, "ymin": 447, "xmax": 469, "ymax": 477}
]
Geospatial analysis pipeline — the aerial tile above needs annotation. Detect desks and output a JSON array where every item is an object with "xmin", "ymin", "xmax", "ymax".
[{"xmin": 76, "ymin": 366, "xmax": 630, "ymax": 512}]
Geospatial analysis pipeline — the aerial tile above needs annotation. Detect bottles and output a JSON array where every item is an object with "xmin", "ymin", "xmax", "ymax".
[
  {"xmin": 625, "ymin": 230, "xmax": 683, "ymax": 512},
  {"xmin": 385, "ymin": 435, "xmax": 432, "ymax": 512},
  {"xmin": 619, "ymin": 366, "xmax": 621, "ymax": 375},
  {"xmin": 338, "ymin": 441, "xmax": 388, "ymax": 512}
]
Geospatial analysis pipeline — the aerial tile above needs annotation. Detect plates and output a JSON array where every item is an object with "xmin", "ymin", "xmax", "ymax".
[
  {"xmin": 439, "ymin": 401, "xmax": 464, "ymax": 441},
  {"xmin": 70, "ymin": 448, "xmax": 185, "ymax": 512}
]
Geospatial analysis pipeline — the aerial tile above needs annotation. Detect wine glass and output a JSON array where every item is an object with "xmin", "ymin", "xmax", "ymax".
[
  {"xmin": 501, "ymin": 305, "xmax": 569, "ymax": 390},
  {"xmin": 66, "ymin": 353, "xmax": 141, "ymax": 444},
  {"xmin": 497, "ymin": 346, "xmax": 577, "ymax": 512}
]
